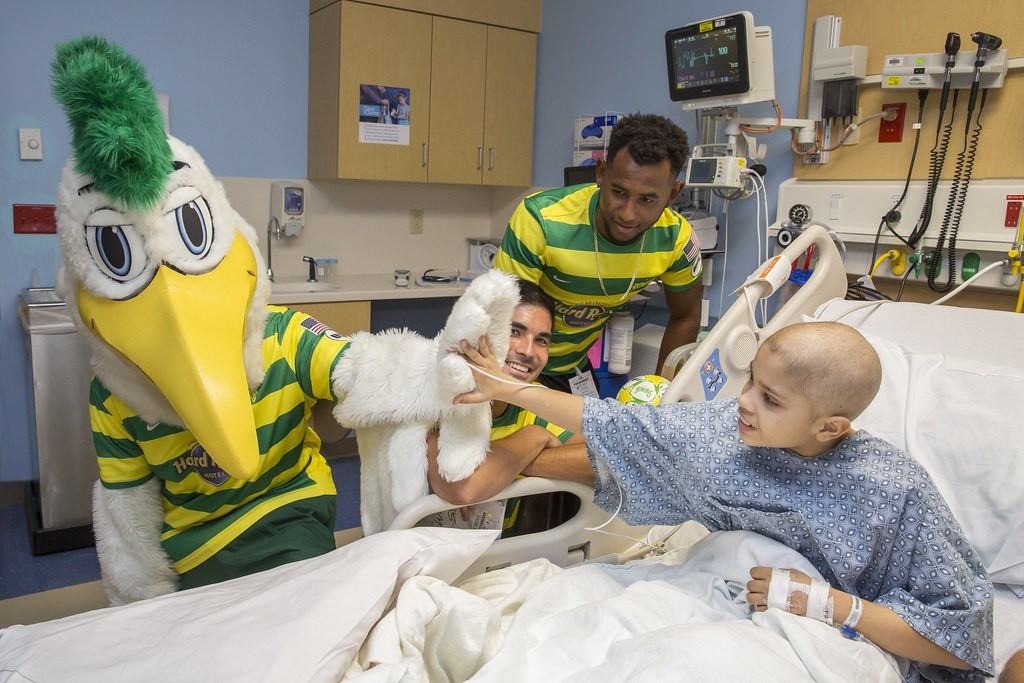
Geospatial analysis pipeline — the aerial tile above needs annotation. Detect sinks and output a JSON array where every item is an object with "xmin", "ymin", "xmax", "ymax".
[{"xmin": 270, "ymin": 283, "xmax": 332, "ymax": 292}]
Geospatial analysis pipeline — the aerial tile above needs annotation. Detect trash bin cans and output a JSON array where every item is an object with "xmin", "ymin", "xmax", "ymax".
[{"xmin": 16, "ymin": 287, "xmax": 97, "ymax": 528}]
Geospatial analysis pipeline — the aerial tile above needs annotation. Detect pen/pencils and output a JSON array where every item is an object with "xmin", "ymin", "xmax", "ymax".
[{"xmin": 453, "ymin": 277, "xmax": 473, "ymax": 282}]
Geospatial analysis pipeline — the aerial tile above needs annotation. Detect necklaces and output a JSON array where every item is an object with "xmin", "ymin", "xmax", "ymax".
[{"xmin": 594, "ymin": 216, "xmax": 646, "ymax": 302}]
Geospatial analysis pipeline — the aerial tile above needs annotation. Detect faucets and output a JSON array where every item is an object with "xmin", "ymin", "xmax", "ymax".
[{"xmin": 266, "ymin": 216, "xmax": 280, "ymax": 282}]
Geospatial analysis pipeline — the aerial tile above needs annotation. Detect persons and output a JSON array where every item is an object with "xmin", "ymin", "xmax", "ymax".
[
  {"xmin": 491, "ymin": 112, "xmax": 702, "ymax": 400},
  {"xmin": 425, "ymin": 278, "xmax": 587, "ymax": 540},
  {"xmin": 447, "ymin": 321, "xmax": 996, "ymax": 683}
]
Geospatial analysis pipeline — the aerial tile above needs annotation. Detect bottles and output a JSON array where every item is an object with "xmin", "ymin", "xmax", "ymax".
[
  {"xmin": 607, "ymin": 306, "xmax": 634, "ymax": 374},
  {"xmin": 315, "ymin": 258, "xmax": 338, "ymax": 283}
]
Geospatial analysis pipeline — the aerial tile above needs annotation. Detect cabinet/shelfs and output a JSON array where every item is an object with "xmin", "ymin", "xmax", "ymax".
[
  {"xmin": 271, "ymin": 296, "xmax": 461, "ymax": 461},
  {"xmin": 309, "ymin": 0, "xmax": 539, "ymax": 187}
]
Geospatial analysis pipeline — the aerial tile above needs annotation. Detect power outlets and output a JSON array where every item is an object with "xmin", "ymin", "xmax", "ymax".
[
  {"xmin": 878, "ymin": 103, "xmax": 906, "ymax": 142},
  {"xmin": 891, "ymin": 194, "xmax": 906, "ymax": 222},
  {"xmin": 409, "ymin": 210, "xmax": 424, "ymax": 234},
  {"xmin": 13, "ymin": 203, "xmax": 56, "ymax": 234}
]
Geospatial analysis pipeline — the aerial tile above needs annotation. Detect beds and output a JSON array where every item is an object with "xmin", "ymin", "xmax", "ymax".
[{"xmin": 391, "ymin": 227, "xmax": 1023, "ymax": 683}]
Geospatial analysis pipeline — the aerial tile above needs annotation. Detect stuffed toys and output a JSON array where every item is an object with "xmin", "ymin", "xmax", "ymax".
[{"xmin": 47, "ymin": 35, "xmax": 522, "ymax": 606}]
